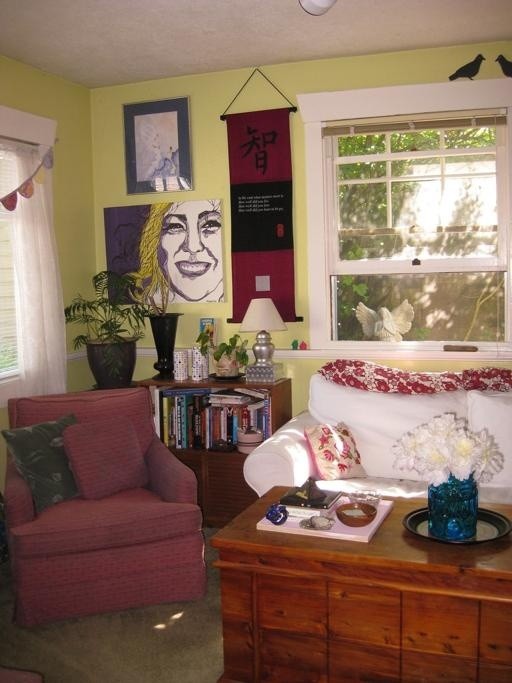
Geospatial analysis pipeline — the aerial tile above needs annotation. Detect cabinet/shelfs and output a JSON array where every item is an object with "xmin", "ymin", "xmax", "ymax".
[{"xmin": 136, "ymin": 375, "xmax": 293, "ymax": 529}]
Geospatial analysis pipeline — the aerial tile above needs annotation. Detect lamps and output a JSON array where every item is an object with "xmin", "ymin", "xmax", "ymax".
[{"xmin": 239, "ymin": 298, "xmax": 288, "ymax": 382}]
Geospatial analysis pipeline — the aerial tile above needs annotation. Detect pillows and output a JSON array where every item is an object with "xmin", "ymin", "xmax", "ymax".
[
  {"xmin": 304, "ymin": 420, "xmax": 367, "ymax": 479},
  {"xmin": 1, "ymin": 412, "xmax": 151, "ymax": 518}
]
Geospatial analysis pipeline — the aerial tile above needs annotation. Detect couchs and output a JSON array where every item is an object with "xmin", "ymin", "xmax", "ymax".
[
  {"xmin": 3, "ymin": 387, "xmax": 207, "ymax": 628},
  {"xmin": 243, "ymin": 358, "xmax": 511, "ymax": 504}
]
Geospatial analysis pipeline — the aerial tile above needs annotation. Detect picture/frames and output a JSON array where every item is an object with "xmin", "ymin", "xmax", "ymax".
[{"xmin": 121, "ymin": 93, "xmax": 195, "ymax": 195}]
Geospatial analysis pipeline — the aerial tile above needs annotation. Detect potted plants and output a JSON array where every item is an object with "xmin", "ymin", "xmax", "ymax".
[
  {"xmin": 64, "ymin": 266, "xmax": 184, "ymax": 390},
  {"xmin": 196, "ymin": 332, "xmax": 249, "ymax": 382}
]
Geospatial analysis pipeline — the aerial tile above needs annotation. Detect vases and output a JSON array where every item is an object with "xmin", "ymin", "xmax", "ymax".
[{"xmin": 427, "ymin": 470, "xmax": 478, "ymax": 539}]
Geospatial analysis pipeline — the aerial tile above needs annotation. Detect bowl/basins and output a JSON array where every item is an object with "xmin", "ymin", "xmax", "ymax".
[
  {"xmin": 348, "ymin": 488, "xmax": 381, "ymax": 505},
  {"xmin": 336, "ymin": 504, "xmax": 377, "ymax": 527}
]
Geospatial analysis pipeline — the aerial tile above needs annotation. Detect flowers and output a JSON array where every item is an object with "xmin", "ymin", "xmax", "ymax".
[{"xmin": 392, "ymin": 412, "xmax": 504, "ymax": 487}]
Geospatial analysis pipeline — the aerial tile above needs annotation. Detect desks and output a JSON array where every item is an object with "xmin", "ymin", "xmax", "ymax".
[{"xmin": 210, "ymin": 485, "xmax": 511, "ymax": 683}]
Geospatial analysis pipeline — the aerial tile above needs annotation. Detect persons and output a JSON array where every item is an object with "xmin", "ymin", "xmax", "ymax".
[
  {"xmin": 374, "ymin": 305, "xmax": 399, "ymax": 342},
  {"xmin": 136, "ymin": 198, "xmax": 225, "ymax": 303}
]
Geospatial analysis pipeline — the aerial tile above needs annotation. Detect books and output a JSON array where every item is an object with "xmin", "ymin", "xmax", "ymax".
[
  {"xmin": 275, "ymin": 485, "xmax": 344, "ymax": 520},
  {"xmin": 146, "ymin": 386, "xmax": 274, "ymax": 454}
]
